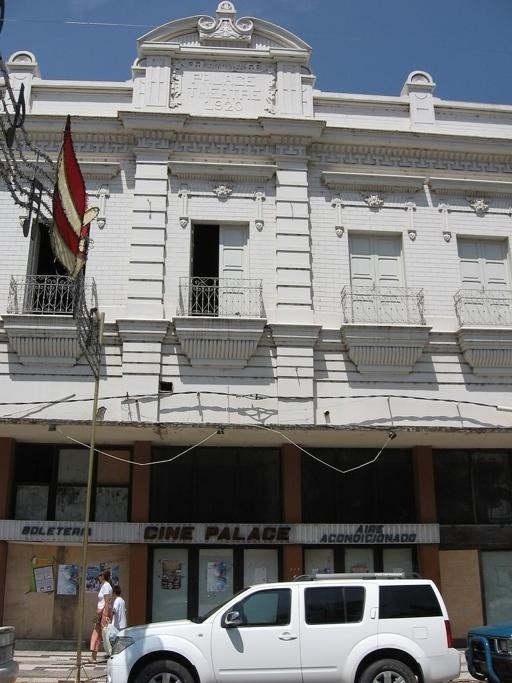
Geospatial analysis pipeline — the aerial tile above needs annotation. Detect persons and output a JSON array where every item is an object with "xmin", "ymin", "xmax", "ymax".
[
  {"xmin": 111, "ymin": 586, "xmax": 127, "ymax": 633},
  {"xmin": 83, "ymin": 570, "xmax": 112, "ymax": 664}
]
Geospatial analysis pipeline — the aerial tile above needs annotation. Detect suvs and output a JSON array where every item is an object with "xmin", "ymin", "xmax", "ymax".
[
  {"xmin": 456, "ymin": 616, "xmax": 511, "ymax": 679},
  {"xmin": 108, "ymin": 568, "xmax": 463, "ymax": 683}
]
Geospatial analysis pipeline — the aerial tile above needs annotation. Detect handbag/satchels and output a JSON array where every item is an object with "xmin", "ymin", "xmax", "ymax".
[{"xmin": 92, "ymin": 611, "xmax": 103, "ymax": 623}]
